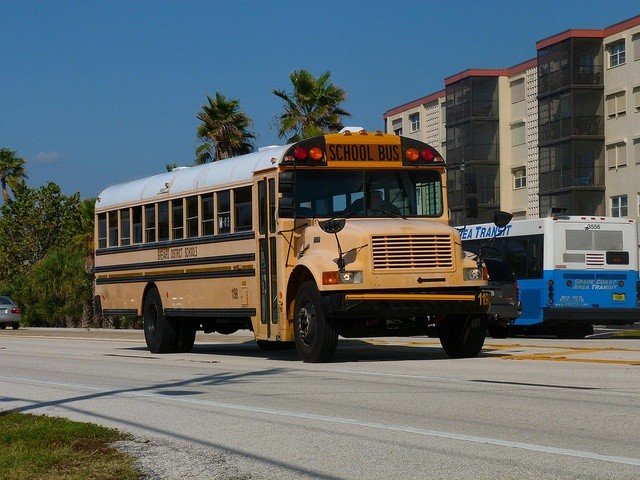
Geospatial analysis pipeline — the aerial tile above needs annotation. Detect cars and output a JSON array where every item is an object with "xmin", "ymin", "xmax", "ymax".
[{"xmin": 0, "ymin": 296, "xmax": 21, "ymax": 330}]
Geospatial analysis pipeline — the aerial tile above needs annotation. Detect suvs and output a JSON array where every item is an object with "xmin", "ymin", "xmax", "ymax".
[{"xmin": 474, "ymin": 256, "xmax": 522, "ymax": 339}]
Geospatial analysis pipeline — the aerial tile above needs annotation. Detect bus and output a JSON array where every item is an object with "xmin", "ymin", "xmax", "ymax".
[
  {"xmin": 94, "ymin": 127, "xmax": 493, "ymax": 365},
  {"xmin": 453, "ymin": 215, "xmax": 640, "ymax": 338}
]
